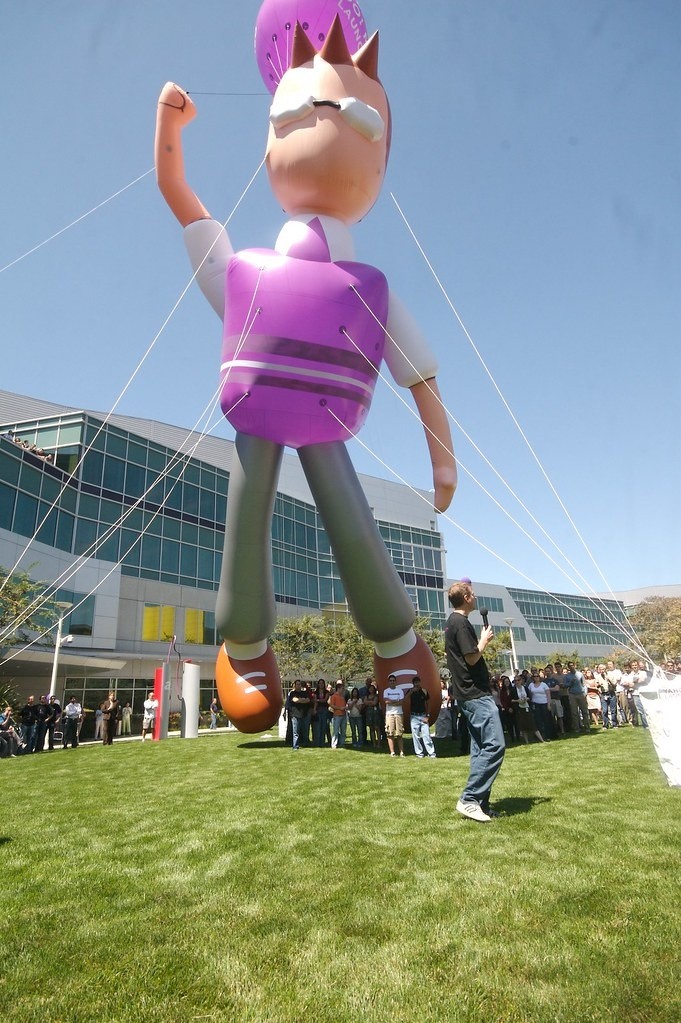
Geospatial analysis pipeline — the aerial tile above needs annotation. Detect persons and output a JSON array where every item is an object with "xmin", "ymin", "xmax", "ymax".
[
  {"xmin": 0, "ymin": 690, "xmax": 133, "ymax": 758},
  {"xmin": 405, "ymin": 677, "xmax": 437, "ymax": 758},
  {"xmin": 446, "ymin": 583, "xmax": 508, "ymax": 822},
  {"xmin": 210, "ymin": 698, "xmax": 218, "ymax": 730},
  {"xmin": 198, "ymin": 711, "xmax": 203, "ymax": 728},
  {"xmin": 283, "ymin": 678, "xmax": 383, "ymax": 752},
  {"xmin": 142, "ymin": 692, "xmax": 158, "ymax": 742},
  {"xmin": 383, "ymin": 675, "xmax": 405, "ymax": 758},
  {"xmin": 490, "ymin": 659, "xmax": 681, "ymax": 745},
  {"xmin": 440, "ymin": 677, "xmax": 455, "ymax": 708}
]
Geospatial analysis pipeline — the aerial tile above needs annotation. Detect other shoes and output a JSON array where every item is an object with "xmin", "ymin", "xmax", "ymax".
[
  {"xmin": 614, "ymin": 727, "xmax": 618, "ymax": 729},
  {"xmin": 603, "ymin": 727, "xmax": 606, "ymax": 731},
  {"xmin": 22, "ymin": 744, "xmax": 27, "ymax": 749},
  {"xmin": 575, "ymin": 730, "xmax": 579, "ymax": 733},
  {"xmin": 10, "ymin": 753, "xmax": 16, "ymax": 757},
  {"xmin": 400, "ymin": 754, "xmax": 405, "ymax": 758},
  {"xmin": 391, "ymin": 753, "xmax": 396, "ymax": 757},
  {"xmin": 586, "ymin": 729, "xmax": 589, "ymax": 733}
]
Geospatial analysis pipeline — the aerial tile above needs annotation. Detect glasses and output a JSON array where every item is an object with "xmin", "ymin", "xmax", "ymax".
[
  {"xmin": 389, "ymin": 680, "xmax": 395, "ymax": 682},
  {"xmin": 514, "ymin": 679, "xmax": 521, "ymax": 682},
  {"xmin": 533, "ymin": 676, "xmax": 539, "ymax": 678}
]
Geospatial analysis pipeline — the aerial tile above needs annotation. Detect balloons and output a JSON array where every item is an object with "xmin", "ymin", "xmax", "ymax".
[{"xmin": 254, "ymin": 0, "xmax": 368, "ymax": 99}]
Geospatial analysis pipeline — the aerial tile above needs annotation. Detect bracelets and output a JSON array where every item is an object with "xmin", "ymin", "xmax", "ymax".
[{"xmin": 426, "ymin": 713, "xmax": 431, "ymax": 717}]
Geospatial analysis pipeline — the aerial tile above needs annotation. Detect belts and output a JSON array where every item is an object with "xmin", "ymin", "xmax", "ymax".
[{"xmin": 411, "ymin": 713, "xmax": 425, "ymax": 716}]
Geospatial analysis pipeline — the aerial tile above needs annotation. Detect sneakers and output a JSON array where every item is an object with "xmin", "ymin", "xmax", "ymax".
[
  {"xmin": 456, "ymin": 801, "xmax": 491, "ymax": 822},
  {"xmin": 486, "ymin": 808, "xmax": 502, "ymax": 817}
]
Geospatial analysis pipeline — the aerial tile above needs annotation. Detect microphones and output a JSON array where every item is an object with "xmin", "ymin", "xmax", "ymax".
[{"xmin": 480, "ymin": 608, "xmax": 488, "ymax": 629}]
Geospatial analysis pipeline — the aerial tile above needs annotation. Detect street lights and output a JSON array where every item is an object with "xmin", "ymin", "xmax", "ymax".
[
  {"xmin": 42, "ymin": 601, "xmax": 74, "ymax": 751},
  {"xmin": 503, "ymin": 617, "xmax": 519, "ymax": 673},
  {"xmin": 507, "ymin": 651, "xmax": 515, "ymax": 677}
]
